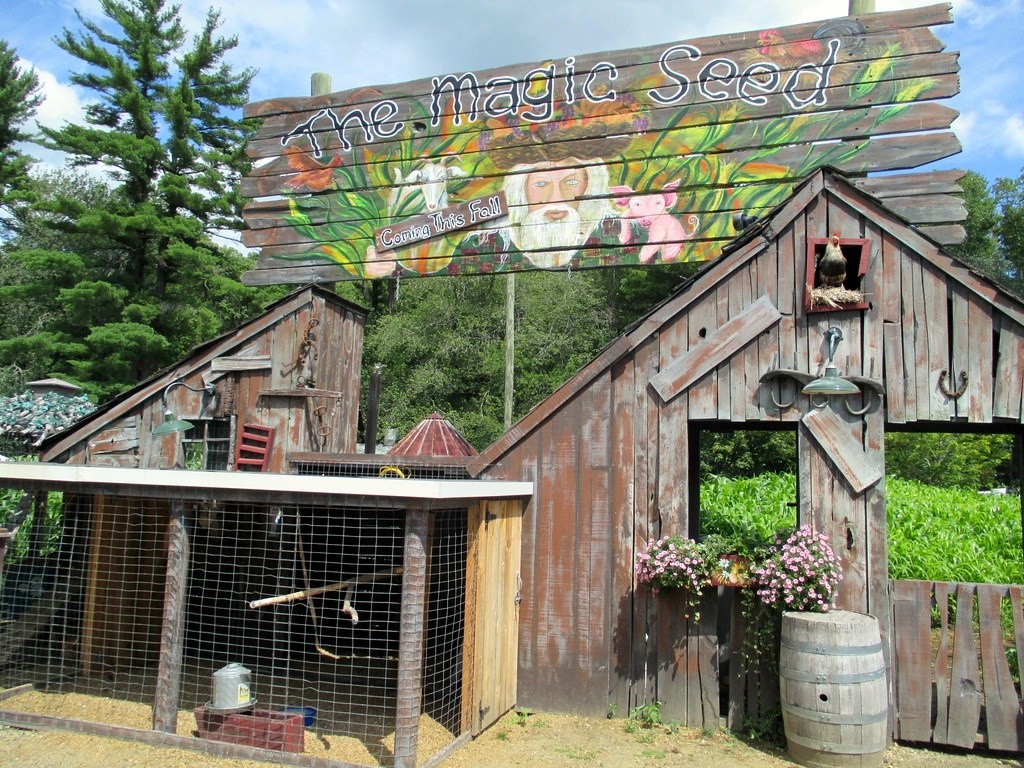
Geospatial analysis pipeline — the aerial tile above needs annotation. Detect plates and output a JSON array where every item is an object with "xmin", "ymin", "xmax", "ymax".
[{"xmin": 205, "ymin": 698, "xmax": 258, "ymax": 714}]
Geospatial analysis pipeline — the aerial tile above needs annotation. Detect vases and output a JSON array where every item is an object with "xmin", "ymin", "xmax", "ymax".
[{"xmin": 681, "ymin": 551, "xmax": 781, "ymax": 590}]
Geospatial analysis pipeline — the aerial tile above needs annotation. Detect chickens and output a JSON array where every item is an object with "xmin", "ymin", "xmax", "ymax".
[{"xmin": 817, "ymin": 231, "xmax": 849, "ymax": 288}]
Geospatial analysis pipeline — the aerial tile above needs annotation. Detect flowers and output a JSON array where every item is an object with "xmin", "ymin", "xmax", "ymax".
[{"xmin": 635, "ymin": 524, "xmax": 847, "ymax": 681}]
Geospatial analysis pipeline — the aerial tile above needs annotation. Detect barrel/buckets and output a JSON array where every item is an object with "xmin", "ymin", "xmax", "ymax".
[{"xmin": 779, "ymin": 610, "xmax": 888, "ymax": 768}]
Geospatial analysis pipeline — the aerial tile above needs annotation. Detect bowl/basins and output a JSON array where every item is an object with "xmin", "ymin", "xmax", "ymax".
[{"xmin": 276, "ymin": 707, "xmax": 317, "ymax": 726}]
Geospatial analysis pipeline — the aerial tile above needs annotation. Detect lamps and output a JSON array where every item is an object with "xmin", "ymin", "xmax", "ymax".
[
  {"xmin": 153, "ymin": 380, "xmax": 217, "ymax": 437},
  {"xmin": 802, "ymin": 327, "xmax": 860, "ymax": 395},
  {"xmin": 732, "ymin": 212, "xmax": 758, "ymax": 231}
]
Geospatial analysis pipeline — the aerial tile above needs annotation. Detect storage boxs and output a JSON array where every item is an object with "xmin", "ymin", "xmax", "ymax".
[{"xmin": 193, "ymin": 701, "xmax": 304, "ymax": 755}]
